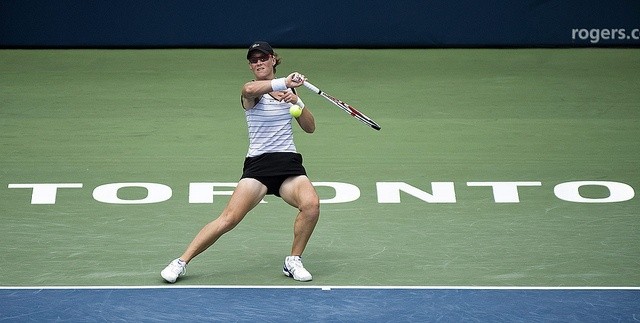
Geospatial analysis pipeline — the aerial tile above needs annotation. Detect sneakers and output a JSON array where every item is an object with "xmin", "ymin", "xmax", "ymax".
[
  {"xmin": 283, "ymin": 255, "xmax": 313, "ymax": 281},
  {"xmin": 161, "ymin": 258, "xmax": 188, "ymax": 282}
]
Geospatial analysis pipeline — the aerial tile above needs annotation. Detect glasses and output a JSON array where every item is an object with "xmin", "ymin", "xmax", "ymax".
[{"xmin": 249, "ymin": 54, "xmax": 269, "ymax": 63}]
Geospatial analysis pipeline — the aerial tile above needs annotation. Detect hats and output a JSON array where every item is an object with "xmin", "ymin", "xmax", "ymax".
[{"xmin": 247, "ymin": 41, "xmax": 274, "ymax": 58}]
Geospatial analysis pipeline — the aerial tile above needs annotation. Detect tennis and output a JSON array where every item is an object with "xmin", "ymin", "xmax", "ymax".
[{"xmin": 289, "ymin": 105, "xmax": 302, "ymax": 118}]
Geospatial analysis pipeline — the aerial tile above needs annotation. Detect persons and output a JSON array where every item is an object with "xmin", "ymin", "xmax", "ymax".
[{"xmin": 161, "ymin": 41, "xmax": 320, "ymax": 283}]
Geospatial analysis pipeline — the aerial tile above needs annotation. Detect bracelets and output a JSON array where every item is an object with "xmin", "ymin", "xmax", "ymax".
[
  {"xmin": 271, "ymin": 77, "xmax": 287, "ymax": 92},
  {"xmin": 293, "ymin": 95, "xmax": 305, "ymax": 109}
]
